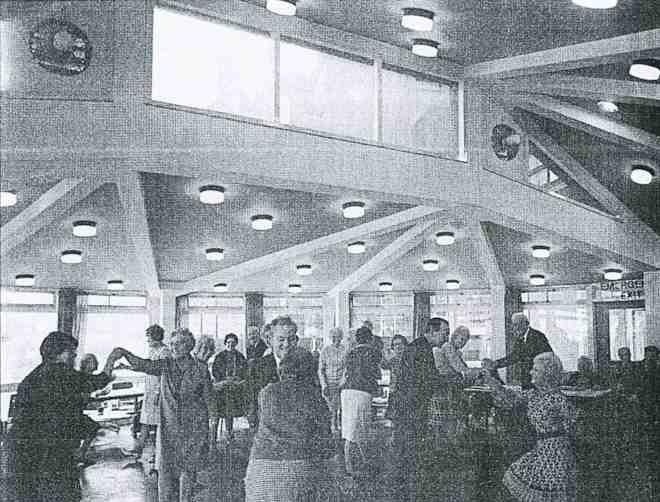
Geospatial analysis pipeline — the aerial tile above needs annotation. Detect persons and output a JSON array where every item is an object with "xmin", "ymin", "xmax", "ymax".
[
  {"xmin": 1, "ymin": 328, "xmax": 124, "ymax": 500},
  {"xmin": 241, "ymin": 346, "xmax": 334, "ymax": 502},
  {"xmin": 482, "ymin": 350, "xmax": 584, "ymax": 502},
  {"xmin": 123, "ymin": 328, "xmax": 214, "ymax": 502},
  {"xmin": 74, "ymin": 313, "xmax": 660, "ymax": 476}
]
